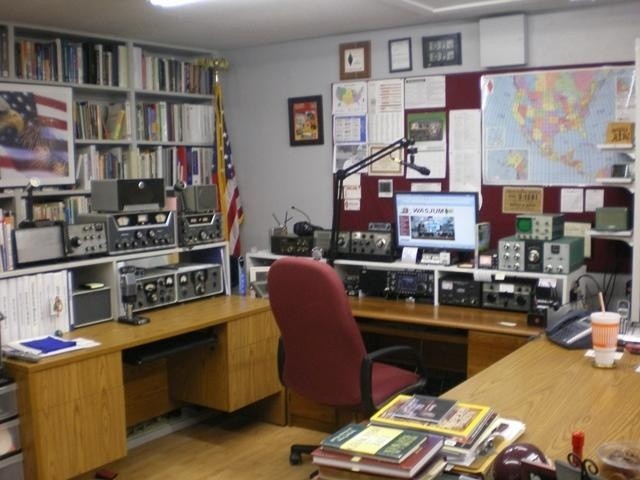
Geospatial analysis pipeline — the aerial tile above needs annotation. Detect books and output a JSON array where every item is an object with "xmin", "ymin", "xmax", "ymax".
[
  {"xmin": 0, "ymin": 35, "xmax": 132, "ymax": 139},
  {"xmin": 75, "ymin": 146, "xmax": 215, "ymax": 190},
  {"xmin": 310, "ymin": 394, "xmax": 528, "ymax": 480},
  {"xmin": 130, "ymin": 47, "xmax": 215, "ymax": 141},
  {"xmin": 0, "ymin": 195, "xmax": 95, "ymax": 227}
]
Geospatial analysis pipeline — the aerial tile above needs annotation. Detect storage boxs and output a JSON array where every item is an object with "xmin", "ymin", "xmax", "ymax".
[{"xmin": 0, "ymin": 374, "xmax": 24, "ymax": 479}]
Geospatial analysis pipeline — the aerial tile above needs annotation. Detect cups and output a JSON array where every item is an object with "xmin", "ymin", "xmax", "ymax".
[{"xmin": 590, "ymin": 311, "xmax": 621, "ymax": 369}]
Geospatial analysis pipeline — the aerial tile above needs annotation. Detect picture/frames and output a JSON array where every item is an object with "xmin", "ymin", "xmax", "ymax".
[
  {"xmin": 389, "ymin": 38, "xmax": 413, "ymax": 72},
  {"xmin": 339, "ymin": 40, "xmax": 371, "ymax": 79},
  {"xmin": 287, "ymin": 95, "xmax": 324, "ymax": 146}
]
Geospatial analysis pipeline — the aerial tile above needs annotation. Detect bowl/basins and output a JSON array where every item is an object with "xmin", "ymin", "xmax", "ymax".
[{"xmin": 593, "ymin": 440, "xmax": 640, "ymax": 479}]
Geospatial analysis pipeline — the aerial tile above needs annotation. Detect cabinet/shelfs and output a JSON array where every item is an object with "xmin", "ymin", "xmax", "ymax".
[
  {"xmin": 589, "ymin": 37, "xmax": 640, "ymax": 321},
  {"xmin": 246, "ymin": 249, "xmax": 330, "ymax": 298},
  {"xmin": 1, "ymin": 20, "xmax": 230, "ymax": 330}
]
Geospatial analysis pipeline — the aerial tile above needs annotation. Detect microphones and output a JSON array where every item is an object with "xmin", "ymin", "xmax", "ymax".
[{"xmin": 391, "ymin": 157, "xmax": 430, "ymax": 175}]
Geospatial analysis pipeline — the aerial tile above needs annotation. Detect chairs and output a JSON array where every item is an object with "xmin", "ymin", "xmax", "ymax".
[{"xmin": 267, "ymin": 258, "xmax": 427, "ymax": 466}]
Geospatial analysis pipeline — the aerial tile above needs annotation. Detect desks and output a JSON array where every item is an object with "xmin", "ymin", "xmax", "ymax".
[
  {"xmin": 0, "ymin": 294, "xmax": 280, "ymax": 479},
  {"xmin": 319, "ymin": 336, "xmax": 640, "ymax": 480},
  {"xmin": 347, "ymin": 294, "xmax": 545, "ymax": 378}
]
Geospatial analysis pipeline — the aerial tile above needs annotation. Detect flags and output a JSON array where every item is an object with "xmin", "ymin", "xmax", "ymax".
[{"xmin": 215, "ymin": 85, "xmax": 245, "ymax": 258}]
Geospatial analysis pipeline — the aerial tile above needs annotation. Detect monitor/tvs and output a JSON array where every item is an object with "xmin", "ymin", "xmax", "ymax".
[{"xmin": 393, "ymin": 191, "xmax": 479, "ymax": 252}]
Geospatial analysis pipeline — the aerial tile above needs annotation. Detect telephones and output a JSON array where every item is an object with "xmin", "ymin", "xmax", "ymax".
[{"xmin": 546, "ymin": 311, "xmax": 592, "ymax": 349}]
